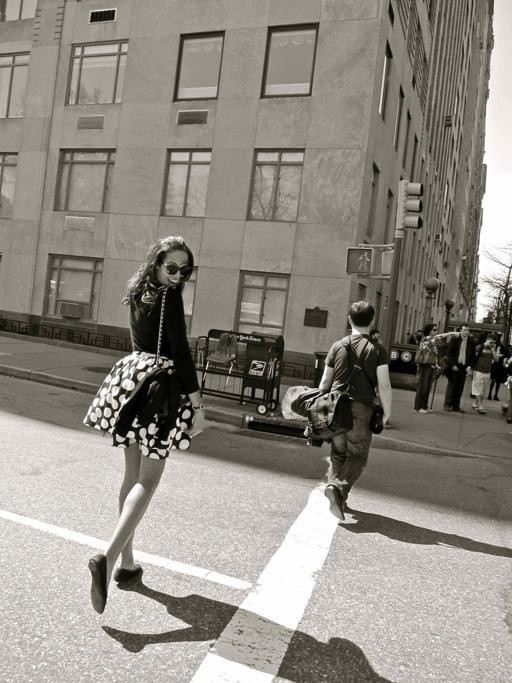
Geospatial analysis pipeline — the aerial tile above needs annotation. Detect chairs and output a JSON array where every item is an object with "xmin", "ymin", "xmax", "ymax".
[{"xmin": 202, "ymin": 332, "xmax": 241, "ymax": 390}]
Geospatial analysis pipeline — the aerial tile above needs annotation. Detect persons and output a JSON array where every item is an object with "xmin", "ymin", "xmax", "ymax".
[
  {"xmin": 317, "ymin": 300, "xmax": 395, "ymax": 521},
  {"xmin": 81, "ymin": 235, "xmax": 207, "ymax": 615},
  {"xmin": 369, "ymin": 322, "xmax": 511, "ymax": 423}
]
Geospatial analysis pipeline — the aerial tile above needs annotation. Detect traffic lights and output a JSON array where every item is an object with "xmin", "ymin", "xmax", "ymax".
[
  {"xmin": 345, "ymin": 247, "xmax": 375, "ymax": 275},
  {"xmin": 396, "ymin": 178, "xmax": 424, "ymax": 228}
]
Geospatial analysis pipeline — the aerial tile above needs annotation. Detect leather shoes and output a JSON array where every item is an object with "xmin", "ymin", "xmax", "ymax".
[{"xmin": 325, "ymin": 484, "xmax": 351, "ymax": 520}]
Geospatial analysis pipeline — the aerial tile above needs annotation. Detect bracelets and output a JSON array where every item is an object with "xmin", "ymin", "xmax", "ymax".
[{"xmin": 191, "ymin": 402, "xmax": 206, "ymax": 411}]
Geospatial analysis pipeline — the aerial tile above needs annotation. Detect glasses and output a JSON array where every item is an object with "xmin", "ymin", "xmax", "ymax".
[
  {"xmin": 164, "ymin": 261, "xmax": 192, "ymax": 275},
  {"xmin": 490, "ymin": 341, "xmax": 496, "ymax": 344},
  {"xmin": 434, "ymin": 329, "xmax": 437, "ymax": 331}
]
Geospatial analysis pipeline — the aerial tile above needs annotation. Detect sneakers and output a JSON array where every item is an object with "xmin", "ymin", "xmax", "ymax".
[{"xmin": 413, "ymin": 405, "xmax": 512, "ymax": 424}]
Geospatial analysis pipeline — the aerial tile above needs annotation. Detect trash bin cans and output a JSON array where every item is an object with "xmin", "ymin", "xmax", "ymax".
[
  {"xmin": 313, "ymin": 351, "xmax": 329, "ymax": 388},
  {"xmin": 240, "ymin": 332, "xmax": 285, "ymax": 406}
]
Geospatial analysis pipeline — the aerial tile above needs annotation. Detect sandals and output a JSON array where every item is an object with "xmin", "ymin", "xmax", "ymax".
[
  {"xmin": 88, "ymin": 554, "xmax": 107, "ymax": 614},
  {"xmin": 114, "ymin": 564, "xmax": 143, "ymax": 582}
]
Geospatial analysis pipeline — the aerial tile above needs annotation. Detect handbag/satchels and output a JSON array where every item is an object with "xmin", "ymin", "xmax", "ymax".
[
  {"xmin": 415, "ymin": 347, "xmax": 437, "ymax": 365},
  {"xmin": 130, "ymin": 366, "xmax": 190, "ymax": 410},
  {"xmin": 370, "ymin": 405, "xmax": 384, "ymax": 434},
  {"xmin": 308, "ymin": 390, "xmax": 353, "ymax": 439}
]
